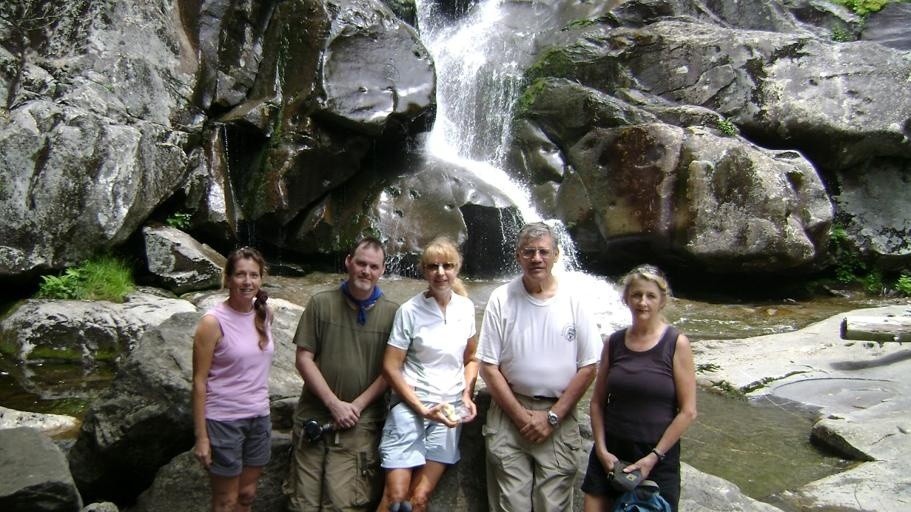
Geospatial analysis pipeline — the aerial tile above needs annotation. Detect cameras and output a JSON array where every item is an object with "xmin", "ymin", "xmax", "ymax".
[{"xmin": 304, "ymin": 417, "xmax": 323, "ymax": 442}]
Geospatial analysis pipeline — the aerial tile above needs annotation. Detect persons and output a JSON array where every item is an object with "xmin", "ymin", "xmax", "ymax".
[
  {"xmin": 377, "ymin": 235, "xmax": 480, "ymax": 512},
  {"xmin": 475, "ymin": 221, "xmax": 605, "ymax": 512},
  {"xmin": 193, "ymin": 244, "xmax": 276, "ymax": 512},
  {"xmin": 287, "ymin": 236, "xmax": 402, "ymax": 512},
  {"xmin": 584, "ymin": 264, "xmax": 699, "ymax": 512}
]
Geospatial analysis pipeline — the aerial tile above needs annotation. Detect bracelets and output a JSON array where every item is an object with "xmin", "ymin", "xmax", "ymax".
[{"xmin": 652, "ymin": 447, "xmax": 666, "ymax": 462}]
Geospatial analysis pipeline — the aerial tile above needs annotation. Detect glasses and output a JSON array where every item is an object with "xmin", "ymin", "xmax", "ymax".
[
  {"xmin": 518, "ymin": 246, "xmax": 553, "ymax": 259},
  {"xmin": 630, "ymin": 267, "xmax": 660, "ymax": 276},
  {"xmin": 422, "ymin": 262, "xmax": 456, "ymax": 270}
]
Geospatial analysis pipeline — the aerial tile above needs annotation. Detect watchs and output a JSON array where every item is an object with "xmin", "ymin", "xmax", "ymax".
[{"xmin": 546, "ymin": 410, "xmax": 560, "ymax": 428}]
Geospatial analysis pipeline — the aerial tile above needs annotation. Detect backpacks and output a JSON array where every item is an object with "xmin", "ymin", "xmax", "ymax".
[{"xmin": 611, "ymin": 480, "xmax": 672, "ymax": 512}]
